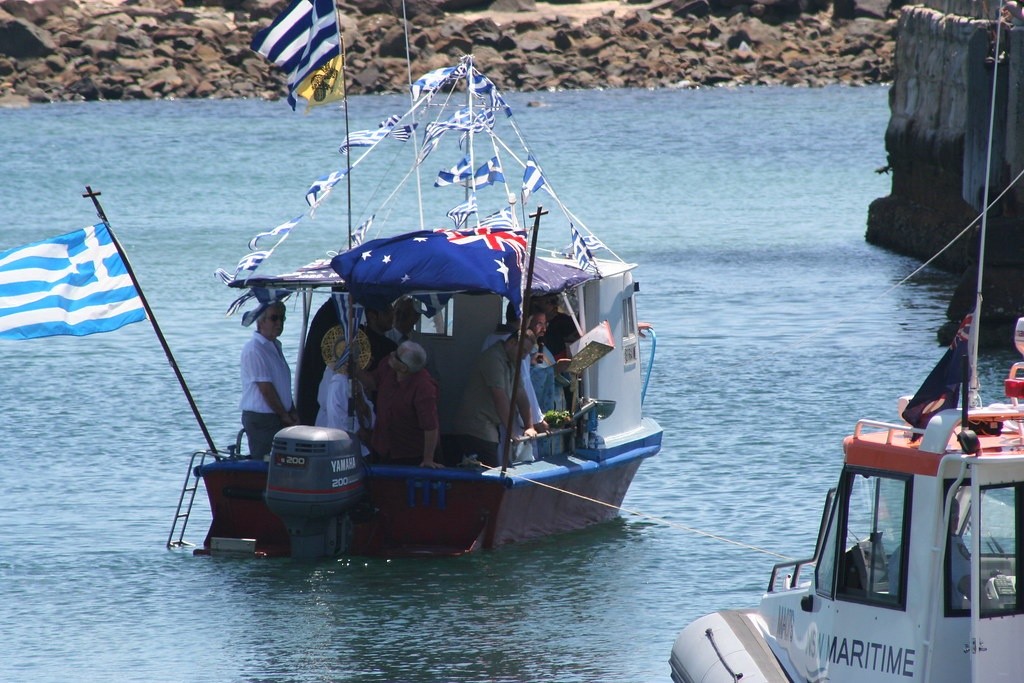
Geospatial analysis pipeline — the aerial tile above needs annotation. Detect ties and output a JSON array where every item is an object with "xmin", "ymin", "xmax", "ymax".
[{"xmin": 402, "ymin": 335, "xmax": 410, "ymax": 342}]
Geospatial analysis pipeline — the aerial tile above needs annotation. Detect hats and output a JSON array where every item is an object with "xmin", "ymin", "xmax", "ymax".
[{"xmin": 321, "ymin": 324, "xmax": 371, "ymax": 376}]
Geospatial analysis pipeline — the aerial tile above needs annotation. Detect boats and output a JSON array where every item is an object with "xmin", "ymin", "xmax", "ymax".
[
  {"xmin": 75, "ymin": 0, "xmax": 664, "ymax": 563},
  {"xmin": 658, "ymin": 0, "xmax": 1024, "ymax": 683}
]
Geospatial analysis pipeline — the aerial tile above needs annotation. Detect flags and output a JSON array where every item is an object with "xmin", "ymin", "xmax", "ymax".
[
  {"xmin": 0, "ymin": 221, "xmax": 151, "ymax": 340},
  {"xmin": 901, "ymin": 302, "xmax": 975, "ymax": 442},
  {"xmin": 250, "ymin": 0, "xmax": 345, "ymax": 117},
  {"xmin": 330, "ymin": 227, "xmax": 527, "ymax": 322}
]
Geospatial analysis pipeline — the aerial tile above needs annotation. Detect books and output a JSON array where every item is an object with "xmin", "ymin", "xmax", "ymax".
[{"xmin": 568, "ymin": 319, "xmax": 615, "ymax": 374}]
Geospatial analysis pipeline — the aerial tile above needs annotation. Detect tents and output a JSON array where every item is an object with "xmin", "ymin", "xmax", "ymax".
[{"xmin": 532, "ymin": 260, "xmax": 607, "ymax": 298}]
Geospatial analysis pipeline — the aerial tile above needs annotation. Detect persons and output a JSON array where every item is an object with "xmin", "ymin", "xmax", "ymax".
[
  {"xmin": 542, "ymin": 295, "xmax": 581, "ymax": 410},
  {"xmin": 342, "ymin": 341, "xmax": 448, "ymax": 470},
  {"xmin": 240, "ymin": 300, "xmax": 301, "ymax": 461},
  {"xmin": 888, "ymin": 498, "xmax": 989, "ymax": 609},
  {"xmin": 468, "ymin": 300, "xmax": 567, "ymax": 466}
]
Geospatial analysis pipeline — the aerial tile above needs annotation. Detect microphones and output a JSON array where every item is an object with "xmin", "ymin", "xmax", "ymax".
[{"xmin": 536, "ymin": 336, "xmax": 546, "ymax": 363}]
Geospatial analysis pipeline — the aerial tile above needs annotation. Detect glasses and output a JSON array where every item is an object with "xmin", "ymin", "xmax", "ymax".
[
  {"xmin": 265, "ymin": 315, "xmax": 286, "ymax": 321},
  {"xmin": 395, "ymin": 349, "xmax": 408, "ymax": 368},
  {"xmin": 534, "ymin": 322, "xmax": 550, "ymax": 329}
]
